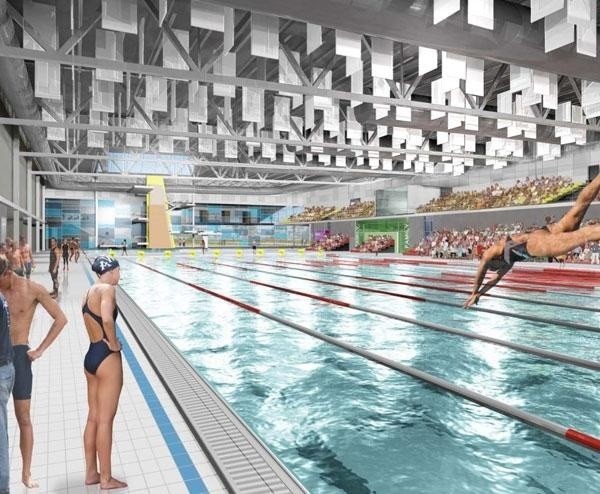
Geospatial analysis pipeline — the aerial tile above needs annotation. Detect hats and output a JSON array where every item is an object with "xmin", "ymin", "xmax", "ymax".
[{"xmin": 92, "ymin": 256, "xmax": 118, "ymax": 274}]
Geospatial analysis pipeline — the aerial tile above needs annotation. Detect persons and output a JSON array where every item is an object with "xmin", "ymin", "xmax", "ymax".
[
  {"xmin": 61, "ymin": 237, "xmax": 80, "ymax": 270},
  {"xmin": 82, "ymin": 256, "xmax": 127, "ymax": 490},
  {"xmin": 252, "ymin": 240, "xmax": 256, "ymax": 255},
  {"xmin": 311, "ymin": 230, "xmax": 349, "ymax": 251},
  {"xmin": 200, "ymin": 240, "xmax": 205, "ymax": 254},
  {"xmin": 463, "ymin": 175, "xmax": 600, "ymax": 310},
  {"xmin": 288, "ymin": 201, "xmax": 374, "ymax": 222},
  {"xmin": 0, "ymin": 235, "xmax": 36, "ymax": 278},
  {"xmin": 418, "ymin": 217, "xmax": 600, "ymax": 266},
  {"xmin": 0, "ymin": 255, "xmax": 68, "ymax": 489},
  {"xmin": 121, "ymin": 239, "xmax": 128, "ymax": 256},
  {"xmin": 355, "ymin": 234, "xmax": 392, "ymax": 252},
  {"xmin": 419, "ymin": 175, "xmax": 573, "ymax": 209},
  {"xmin": 0, "ymin": 290, "xmax": 16, "ymax": 493},
  {"xmin": 49, "ymin": 238, "xmax": 60, "ymax": 299}
]
